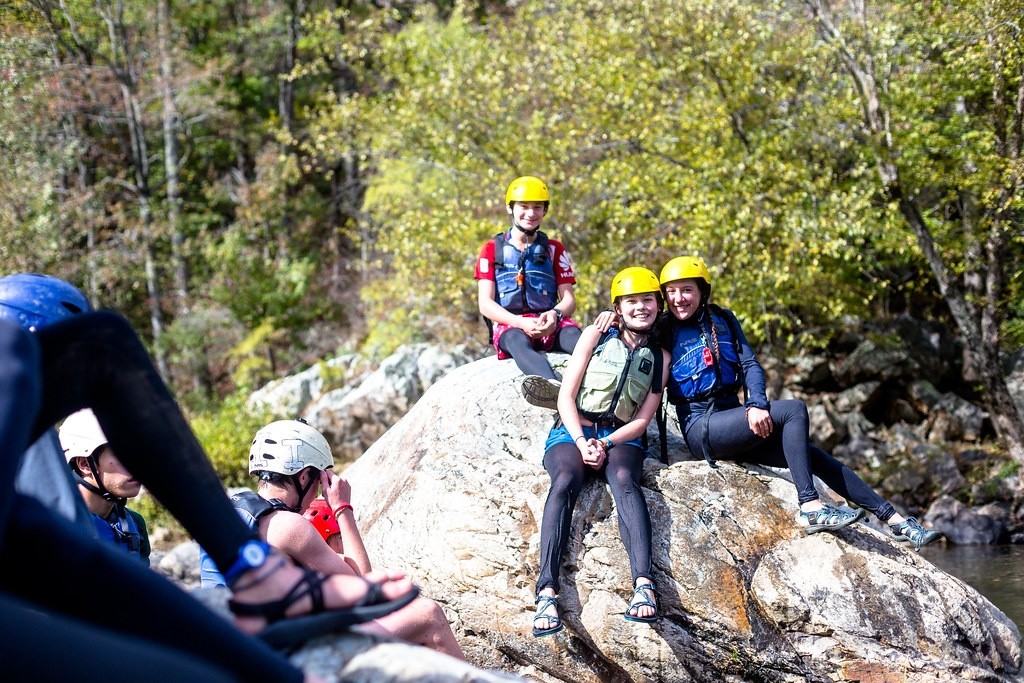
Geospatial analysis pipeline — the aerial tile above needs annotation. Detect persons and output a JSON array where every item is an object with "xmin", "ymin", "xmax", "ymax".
[
  {"xmin": 595, "ymin": 257, "xmax": 944, "ymax": 553},
  {"xmin": 531, "ymin": 268, "xmax": 673, "ymax": 636},
  {"xmin": 0, "ymin": 267, "xmax": 472, "ymax": 683},
  {"xmin": 473, "ymin": 176, "xmax": 583, "ymax": 411}
]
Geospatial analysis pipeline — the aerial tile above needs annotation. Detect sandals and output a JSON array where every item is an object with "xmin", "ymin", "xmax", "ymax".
[
  {"xmin": 228, "ymin": 553, "xmax": 420, "ymax": 659},
  {"xmin": 625, "ymin": 584, "xmax": 658, "ymax": 622},
  {"xmin": 799, "ymin": 503, "xmax": 866, "ymax": 535},
  {"xmin": 532, "ymin": 589, "xmax": 562, "ymax": 637},
  {"xmin": 888, "ymin": 515, "xmax": 945, "ymax": 554}
]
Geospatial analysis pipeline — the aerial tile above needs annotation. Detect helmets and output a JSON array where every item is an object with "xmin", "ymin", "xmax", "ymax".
[
  {"xmin": 0, "ymin": 273, "xmax": 92, "ymax": 333},
  {"xmin": 505, "ymin": 176, "xmax": 550, "ymax": 218},
  {"xmin": 58, "ymin": 407, "xmax": 109, "ymax": 466},
  {"xmin": 302, "ymin": 497, "xmax": 341, "ymax": 543},
  {"xmin": 247, "ymin": 419, "xmax": 335, "ymax": 477},
  {"xmin": 611, "ymin": 266, "xmax": 665, "ymax": 308},
  {"xmin": 659, "ymin": 256, "xmax": 714, "ymax": 306}
]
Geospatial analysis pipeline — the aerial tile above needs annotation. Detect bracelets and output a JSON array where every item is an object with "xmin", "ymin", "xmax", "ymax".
[
  {"xmin": 335, "ymin": 508, "xmax": 350, "ymax": 522},
  {"xmin": 745, "ymin": 407, "xmax": 751, "ymax": 419},
  {"xmin": 333, "ymin": 502, "xmax": 353, "ymax": 516},
  {"xmin": 575, "ymin": 435, "xmax": 585, "ymax": 445},
  {"xmin": 598, "ymin": 439, "xmax": 606, "ymax": 451}
]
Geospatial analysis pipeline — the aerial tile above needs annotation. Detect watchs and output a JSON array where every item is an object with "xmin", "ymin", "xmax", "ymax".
[
  {"xmin": 602, "ymin": 438, "xmax": 613, "ymax": 449},
  {"xmin": 552, "ymin": 308, "xmax": 563, "ymax": 324}
]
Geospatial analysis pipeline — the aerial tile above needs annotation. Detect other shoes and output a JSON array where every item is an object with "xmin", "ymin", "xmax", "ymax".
[{"xmin": 521, "ymin": 375, "xmax": 563, "ymax": 411}]
